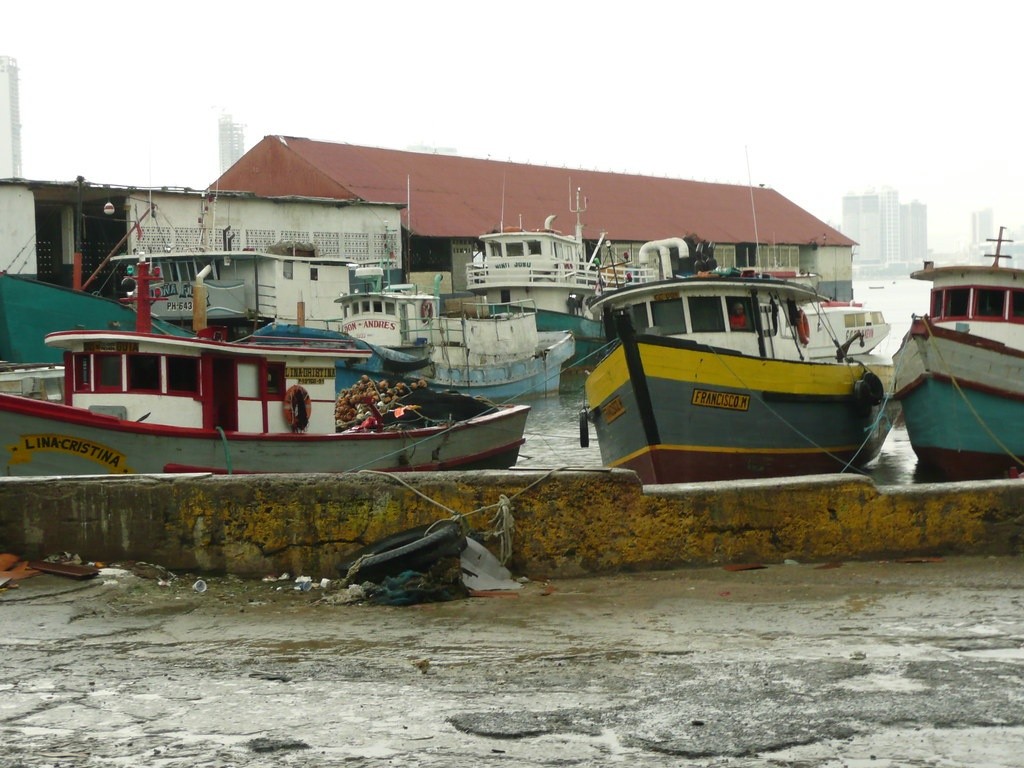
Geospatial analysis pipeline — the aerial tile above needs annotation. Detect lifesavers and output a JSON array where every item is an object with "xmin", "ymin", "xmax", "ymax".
[
  {"xmin": 421, "ymin": 301, "xmax": 434, "ymax": 324},
  {"xmin": 283, "ymin": 385, "xmax": 312, "ymax": 428},
  {"xmin": 567, "ymin": 259, "xmax": 573, "ymax": 269},
  {"xmin": 504, "ymin": 226, "xmax": 521, "ymax": 232},
  {"xmin": 798, "ymin": 309, "xmax": 810, "ymax": 345}
]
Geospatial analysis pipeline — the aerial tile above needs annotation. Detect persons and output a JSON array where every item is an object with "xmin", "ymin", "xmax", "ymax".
[{"xmin": 730, "ymin": 303, "xmax": 747, "ymax": 330}]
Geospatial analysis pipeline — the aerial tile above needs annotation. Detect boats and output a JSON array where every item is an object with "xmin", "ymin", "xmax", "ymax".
[
  {"xmin": 0, "ymin": 173, "xmax": 652, "ymax": 472},
  {"xmin": 572, "ymin": 231, "xmax": 904, "ymax": 484},
  {"xmin": 894, "ymin": 224, "xmax": 1024, "ymax": 480}
]
